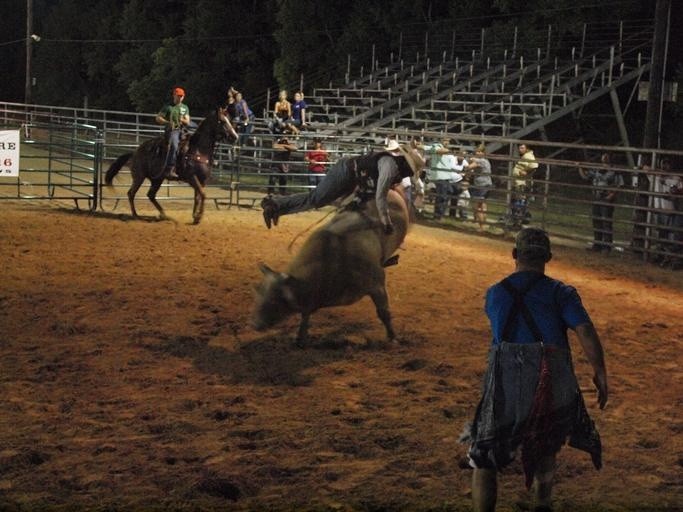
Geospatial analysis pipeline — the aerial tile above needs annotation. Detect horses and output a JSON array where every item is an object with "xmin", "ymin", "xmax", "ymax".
[{"xmin": 104, "ymin": 104, "xmax": 240, "ymax": 225}]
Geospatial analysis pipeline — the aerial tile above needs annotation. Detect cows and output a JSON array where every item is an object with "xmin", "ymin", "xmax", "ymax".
[{"xmin": 249, "ymin": 183, "xmax": 411, "ymax": 350}]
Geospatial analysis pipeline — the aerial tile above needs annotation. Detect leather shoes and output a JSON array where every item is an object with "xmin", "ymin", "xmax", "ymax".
[{"xmin": 261, "ymin": 196, "xmax": 279, "ymax": 229}]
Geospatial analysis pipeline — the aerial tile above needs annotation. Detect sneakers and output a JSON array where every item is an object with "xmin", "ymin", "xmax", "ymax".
[{"xmin": 167, "ymin": 166, "xmax": 178, "ymax": 178}]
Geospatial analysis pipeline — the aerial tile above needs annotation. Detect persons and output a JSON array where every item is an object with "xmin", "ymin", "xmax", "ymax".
[
  {"xmin": 574, "ymin": 150, "xmax": 625, "ymax": 252},
  {"xmin": 384, "ymin": 134, "xmax": 493, "ymax": 233},
  {"xmin": 466, "ymin": 227, "xmax": 608, "ymax": 512},
  {"xmin": 155, "ymin": 87, "xmax": 190, "ymax": 178},
  {"xmin": 267, "ymin": 135, "xmax": 298, "ymax": 198},
  {"xmin": 280, "ymin": 90, "xmax": 308, "ymax": 141},
  {"xmin": 260, "ymin": 147, "xmax": 427, "ymax": 236},
  {"xmin": 220, "ymin": 86, "xmax": 255, "ymax": 151},
  {"xmin": 304, "ymin": 138, "xmax": 329, "ymax": 211},
  {"xmin": 642, "ymin": 158, "xmax": 683, "ymax": 270},
  {"xmin": 510, "ymin": 143, "xmax": 538, "ymax": 227},
  {"xmin": 267, "ymin": 90, "xmax": 291, "ymax": 139}
]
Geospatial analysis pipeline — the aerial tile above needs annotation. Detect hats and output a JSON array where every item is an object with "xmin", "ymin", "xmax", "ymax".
[{"xmin": 173, "ymin": 88, "xmax": 185, "ymax": 96}]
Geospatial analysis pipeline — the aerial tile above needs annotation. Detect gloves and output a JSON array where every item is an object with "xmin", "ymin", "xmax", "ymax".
[
  {"xmin": 384, "ymin": 140, "xmax": 426, "ymax": 183},
  {"xmin": 384, "ymin": 223, "xmax": 394, "ymax": 235}
]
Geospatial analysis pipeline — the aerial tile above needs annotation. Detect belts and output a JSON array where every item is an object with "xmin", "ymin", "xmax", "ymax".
[{"xmin": 350, "ymin": 158, "xmax": 359, "ymax": 183}]
